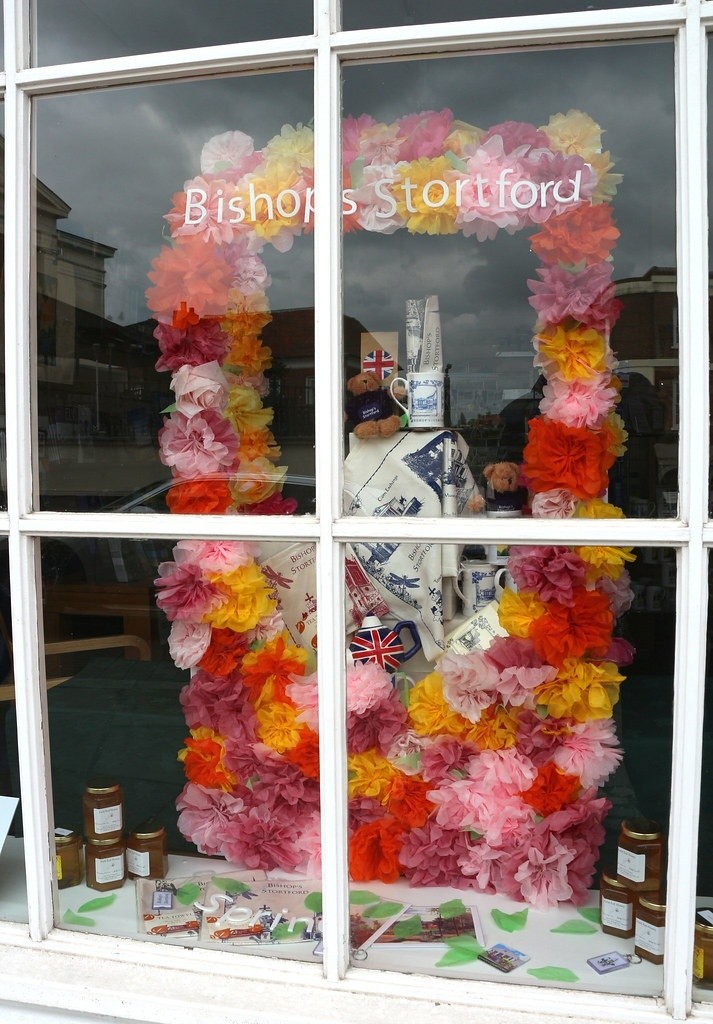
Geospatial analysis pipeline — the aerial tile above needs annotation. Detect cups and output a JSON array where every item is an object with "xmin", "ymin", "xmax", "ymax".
[
  {"xmin": 391, "ymin": 371, "xmax": 445, "ymax": 428},
  {"xmin": 480, "ymin": 510, "xmax": 523, "ymax": 565},
  {"xmin": 453, "ymin": 559, "xmax": 518, "ymax": 618}
]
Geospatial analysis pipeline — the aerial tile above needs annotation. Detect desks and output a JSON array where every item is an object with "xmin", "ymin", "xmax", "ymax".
[{"xmin": 0, "ymin": 828, "xmax": 712, "ymax": 1001}]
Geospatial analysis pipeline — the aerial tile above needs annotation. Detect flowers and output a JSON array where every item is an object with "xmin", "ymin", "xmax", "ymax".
[{"xmin": 147, "ymin": 111, "xmax": 626, "ymax": 917}]
